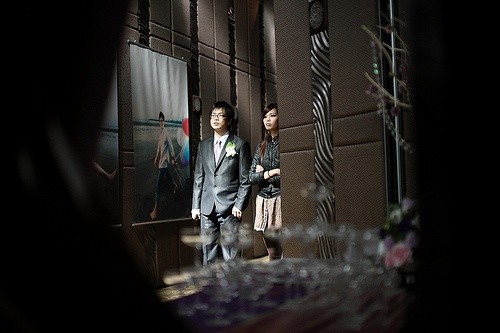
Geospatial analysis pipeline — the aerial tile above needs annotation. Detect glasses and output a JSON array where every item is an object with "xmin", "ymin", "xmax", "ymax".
[{"xmin": 209, "ymin": 114, "xmax": 227, "ymax": 119}]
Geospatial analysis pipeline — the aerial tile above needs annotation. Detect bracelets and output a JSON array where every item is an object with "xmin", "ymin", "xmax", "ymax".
[{"xmin": 268, "ymin": 171, "xmax": 271, "ymax": 178}]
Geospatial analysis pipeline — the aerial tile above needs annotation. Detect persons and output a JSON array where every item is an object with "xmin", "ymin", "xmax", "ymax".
[
  {"xmin": 150, "ymin": 111, "xmax": 180, "ymax": 218},
  {"xmin": 249, "ymin": 103, "xmax": 282, "ymax": 261},
  {"xmin": 91, "ymin": 161, "xmax": 115, "ymax": 182},
  {"xmin": 191, "ymin": 101, "xmax": 251, "ymax": 268}
]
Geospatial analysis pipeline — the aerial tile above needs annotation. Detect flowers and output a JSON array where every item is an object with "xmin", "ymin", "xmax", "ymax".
[
  {"xmin": 225, "ymin": 142, "xmax": 239, "ymax": 157},
  {"xmin": 379, "ymin": 194, "xmax": 421, "ymax": 287},
  {"xmin": 354, "ymin": 13, "xmax": 413, "ymax": 154}
]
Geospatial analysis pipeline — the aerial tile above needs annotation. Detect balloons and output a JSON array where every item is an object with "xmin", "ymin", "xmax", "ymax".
[{"xmin": 176, "ymin": 118, "xmax": 189, "ymax": 165}]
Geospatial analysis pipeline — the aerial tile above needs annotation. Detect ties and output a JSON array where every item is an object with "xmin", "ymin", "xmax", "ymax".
[{"xmin": 213, "ymin": 141, "xmax": 222, "ymax": 166}]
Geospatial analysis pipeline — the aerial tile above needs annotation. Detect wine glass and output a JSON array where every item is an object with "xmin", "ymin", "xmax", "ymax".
[{"xmin": 181, "ymin": 225, "xmax": 379, "ymax": 263}]
